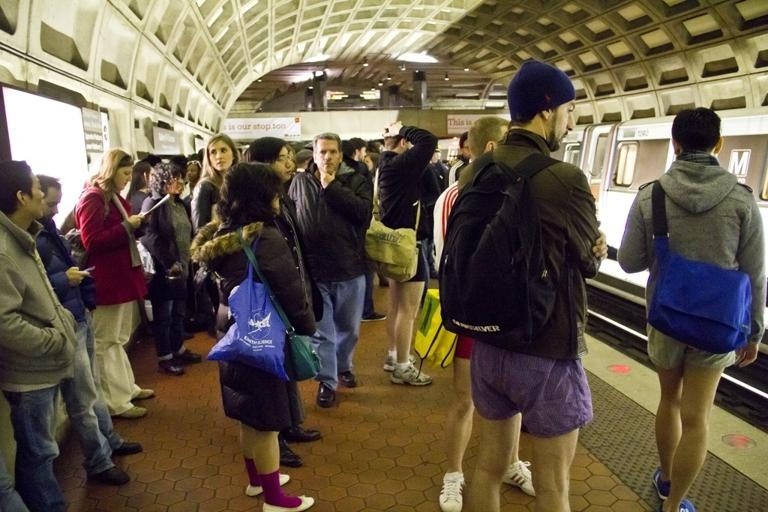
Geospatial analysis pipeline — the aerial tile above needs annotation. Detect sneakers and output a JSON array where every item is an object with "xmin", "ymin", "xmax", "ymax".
[
  {"xmin": 679, "ymin": 500, "xmax": 696, "ymax": 512},
  {"xmin": 261, "ymin": 496, "xmax": 316, "ymax": 511},
  {"xmin": 438, "ymin": 471, "xmax": 465, "ymax": 512},
  {"xmin": 384, "ymin": 352, "xmax": 416, "ymax": 371},
  {"xmin": 391, "ymin": 363, "xmax": 433, "ymax": 386},
  {"xmin": 245, "ymin": 473, "xmax": 291, "ymax": 496},
  {"xmin": 361, "ymin": 311, "xmax": 387, "ymax": 322},
  {"xmin": 653, "ymin": 465, "xmax": 670, "ymax": 499},
  {"xmin": 159, "ymin": 360, "xmax": 184, "ymax": 376},
  {"xmin": 136, "ymin": 389, "xmax": 154, "ymax": 399},
  {"xmin": 176, "ymin": 350, "xmax": 202, "ymax": 364},
  {"xmin": 502, "ymin": 461, "xmax": 537, "ymax": 495},
  {"xmin": 120, "ymin": 406, "xmax": 147, "ymax": 419}
]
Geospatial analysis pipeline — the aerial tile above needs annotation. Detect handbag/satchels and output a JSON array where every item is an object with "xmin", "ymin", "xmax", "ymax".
[
  {"xmin": 240, "ymin": 239, "xmax": 322, "ymax": 382},
  {"xmin": 156, "ymin": 205, "xmax": 189, "ymax": 290},
  {"xmin": 413, "ymin": 288, "xmax": 461, "ymax": 368},
  {"xmin": 206, "ymin": 235, "xmax": 291, "ymax": 382},
  {"xmin": 365, "ymin": 168, "xmax": 421, "ymax": 283},
  {"xmin": 647, "ymin": 181, "xmax": 753, "ymax": 352}
]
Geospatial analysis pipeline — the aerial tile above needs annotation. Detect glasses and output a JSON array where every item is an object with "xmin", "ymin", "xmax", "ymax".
[
  {"xmin": 463, "ymin": 146, "xmax": 469, "ymax": 149},
  {"xmin": 186, "ymin": 159, "xmax": 203, "ymax": 169},
  {"xmin": 277, "ymin": 155, "xmax": 291, "ymax": 162}
]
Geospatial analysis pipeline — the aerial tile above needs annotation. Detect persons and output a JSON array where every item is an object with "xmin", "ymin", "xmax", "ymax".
[{"xmin": 616, "ymin": 107, "xmax": 767, "ymax": 512}]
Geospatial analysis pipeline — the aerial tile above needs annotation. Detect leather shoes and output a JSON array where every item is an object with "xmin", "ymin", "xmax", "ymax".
[
  {"xmin": 278, "ymin": 444, "xmax": 303, "ymax": 467},
  {"xmin": 112, "ymin": 442, "xmax": 142, "ymax": 457},
  {"xmin": 316, "ymin": 381, "xmax": 335, "ymax": 408},
  {"xmin": 285, "ymin": 427, "xmax": 321, "ymax": 443},
  {"xmin": 91, "ymin": 466, "xmax": 129, "ymax": 486},
  {"xmin": 339, "ymin": 371, "xmax": 357, "ymax": 387}
]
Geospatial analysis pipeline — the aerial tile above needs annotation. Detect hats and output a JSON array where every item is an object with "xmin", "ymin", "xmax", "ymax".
[
  {"xmin": 507, "ymin": 58, "xmax": 575, "ymax": 123},
  {"xmin": 296, "ymin": 149, "xmax": 314, "ymax": 164}
]
